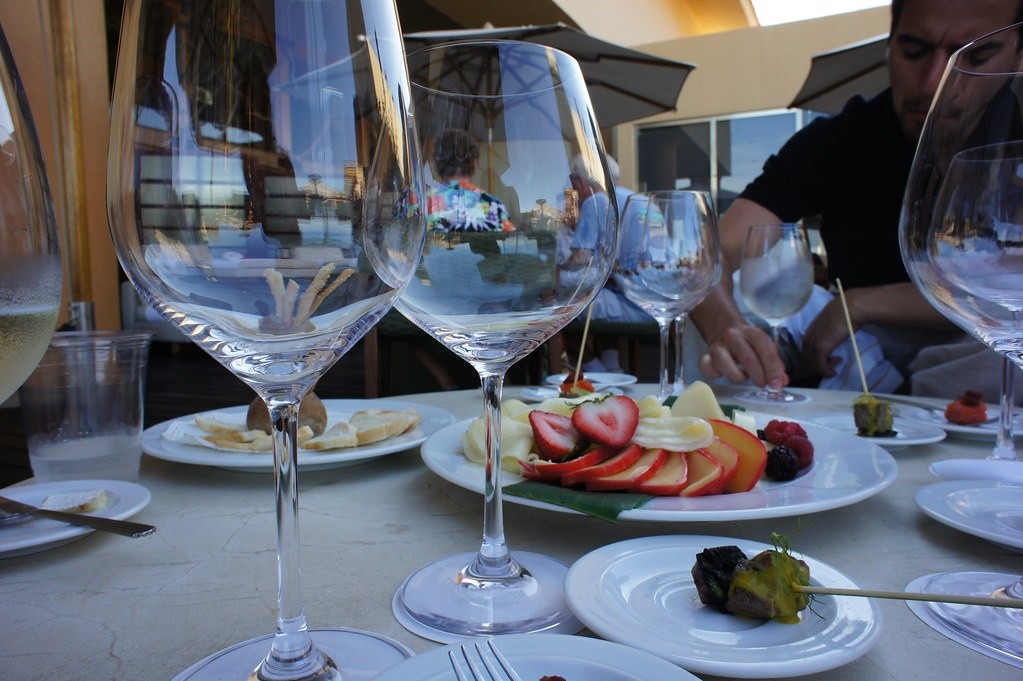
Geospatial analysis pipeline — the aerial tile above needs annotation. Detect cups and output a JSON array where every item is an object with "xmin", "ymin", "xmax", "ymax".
[{"xmin": 16, "ymin": 329, "xmax": 152, "ymax": 485}]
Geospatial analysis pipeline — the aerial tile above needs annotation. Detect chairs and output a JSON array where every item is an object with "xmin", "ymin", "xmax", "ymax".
[{"xmin": 122, "ymin": 149, "xmax": 676, "ymax": 397}]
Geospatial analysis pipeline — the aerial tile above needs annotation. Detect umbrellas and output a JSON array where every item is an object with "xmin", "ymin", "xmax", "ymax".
[
  {"xmin": 787, "ymin": 33, "xmax": 891, "ymax": 114},
  {"xmin": 271, "ymin": 22, "xmax": 697, "ymax": 195}
]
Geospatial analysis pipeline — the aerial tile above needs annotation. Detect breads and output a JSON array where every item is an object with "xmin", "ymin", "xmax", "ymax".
[{"xmin": 194, "ymin": 407, "xmax": 418, "ymax": 452}]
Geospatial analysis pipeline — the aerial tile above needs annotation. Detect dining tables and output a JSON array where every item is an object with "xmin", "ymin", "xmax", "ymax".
[{"xmin": 0, "ymin": 382, "xmax": 1023, "ymax": 680}]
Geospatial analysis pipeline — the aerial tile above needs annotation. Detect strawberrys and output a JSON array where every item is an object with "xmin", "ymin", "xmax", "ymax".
[{"xmin": 528, "ymin": 392, "xmax": 641, "ymax": 460}]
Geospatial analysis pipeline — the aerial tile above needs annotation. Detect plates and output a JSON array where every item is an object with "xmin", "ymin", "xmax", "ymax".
[
  {"xmin": 901, "ymin": 407, "xmax": 1023, "ymax": 443},
  {"xmin": 143, "ymin": 400, "xmax": 455, "ymax": 474},
  {"xmin": 420, "ymin": 406, "xmax": 899, "ymax": 523},
  {"xmin": 0, "ymin": 480, "xmax": 152, "ymax": 561},
  {"xmin": 365, "ymin": 631, "xmax": 700, "ymax": 681},
  {"xmin": 795, "ymin": 414, "xmax": 946, "ymax": 452},
  {"xmin": 546, "ymin": 373, "xmax": 639, "ymax": 386},
  {"xmin": 912, "ymin": 479, "xmax": 1023, "ymax": 549},
  {"xmin": 562, "ymin": 534, "xmax": 881, "ymax": 680},
  {"xmin": 927, "ymin": 459, "xmax": 1023, "ymax": 479}
]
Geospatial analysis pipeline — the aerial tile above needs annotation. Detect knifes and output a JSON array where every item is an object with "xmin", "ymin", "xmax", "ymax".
[{"xmin": 0, "ymin": 496, "xmax": 157, "ymax": 540}]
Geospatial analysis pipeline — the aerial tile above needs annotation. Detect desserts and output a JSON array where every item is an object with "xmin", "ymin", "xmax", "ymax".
[
  {"xmin": 259, "ymin": 262, "xmax": 354, "ymax": 335},
  {"xmin": 248, "ymin": 386, "xmax": 328, "ymax": 435}
]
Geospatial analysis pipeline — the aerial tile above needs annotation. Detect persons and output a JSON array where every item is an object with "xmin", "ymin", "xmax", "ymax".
[
  {"xmin": 682, "ymin": 0, "xmax": 1023, "ymax": 407},
  {"xmin": 396, "ymin": 130, "xmax": 509, "ymax": 234},
  {"xmin": 545, "ymin": 151, "xmax": 674, "ymax": 374}
]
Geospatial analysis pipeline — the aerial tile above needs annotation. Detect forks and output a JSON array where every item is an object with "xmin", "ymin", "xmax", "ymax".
[
  {"xmin": 859, "ymin": 393, "xmax": 1020, "ymax": 425},
  {"xmin": 446, "ymin": 640, "xmax": 522, "ymax": 681}
]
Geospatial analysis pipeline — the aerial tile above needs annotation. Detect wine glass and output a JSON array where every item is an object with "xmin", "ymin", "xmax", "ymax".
[
  {"xmin": 107, "ymin": 0, "xmax": 429, "ymax": 681},
  {"xmin": 607, "ymin": 190, "xmax": 725, "ymax": 395},
  {"xmin": 897, "ymin": 24, "xmax": 1023, "ymax": 668},
  {"xmin": 741, "ymin": 220, "xmax": 814, "ymax": 402},
  {"xmin": 0, "ymin": 28, "xmax": 65, "ymax": 405},
  {"xmin": 371, "ymin": 41, "xmax": 623, "ymax": 647}
]
